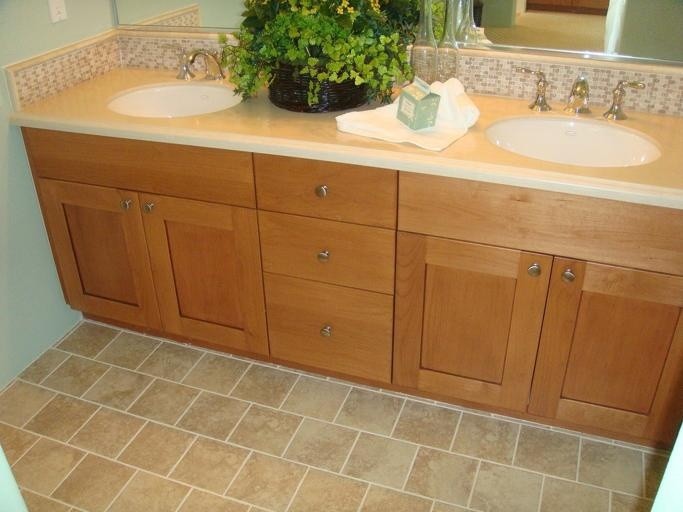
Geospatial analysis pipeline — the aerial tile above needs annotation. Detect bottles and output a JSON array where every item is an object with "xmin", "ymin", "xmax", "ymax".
[
  {"xmin": 410, "ymin": 0, "xmax": 466, "ymax": 89},
  {"xmin": 454, "ymin": 2, "xmax": 479, "ymax": 44}
]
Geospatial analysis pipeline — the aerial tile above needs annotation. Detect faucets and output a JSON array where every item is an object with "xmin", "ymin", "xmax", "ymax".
[
  {"xmin": 188, "ymin": 50, "xmax": 226, "ymax": 81},
  {"xmin": 563, "ymin": 77, "xmax": 591, "ymax": 116}
]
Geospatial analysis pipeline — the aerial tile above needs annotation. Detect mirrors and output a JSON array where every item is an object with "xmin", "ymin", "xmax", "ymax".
[
  {"xmin": 452, "ymin": 0, "xmax": 683, "ymax": 63},
  {"xmin": 112, "ymin": 0, "xmax": 249, "ymax": 33}
]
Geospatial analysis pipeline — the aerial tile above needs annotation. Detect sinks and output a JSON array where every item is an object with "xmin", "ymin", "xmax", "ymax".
[
  {"xmin": 483, "ymin": 113, "xmax": 663, "ymax": 167},
  {"xmin": 106, "ymin": 81, "xmax": 242, "ymax": 118}
]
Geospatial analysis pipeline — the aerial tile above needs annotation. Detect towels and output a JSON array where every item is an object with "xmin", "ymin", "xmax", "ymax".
[{"xmin": 334, "ymin": 76, "xmax": 480, "ymax": 152}]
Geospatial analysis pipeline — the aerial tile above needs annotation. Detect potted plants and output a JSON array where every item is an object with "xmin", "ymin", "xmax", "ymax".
[{"xmin": 219, "ymin": 0, "xmax": 450, "ymax": 111}]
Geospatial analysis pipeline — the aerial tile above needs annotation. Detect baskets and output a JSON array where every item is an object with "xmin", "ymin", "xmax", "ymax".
[{"xmin": 264, "ymin": 64, "xmax": 370, "ymax": 115}]
